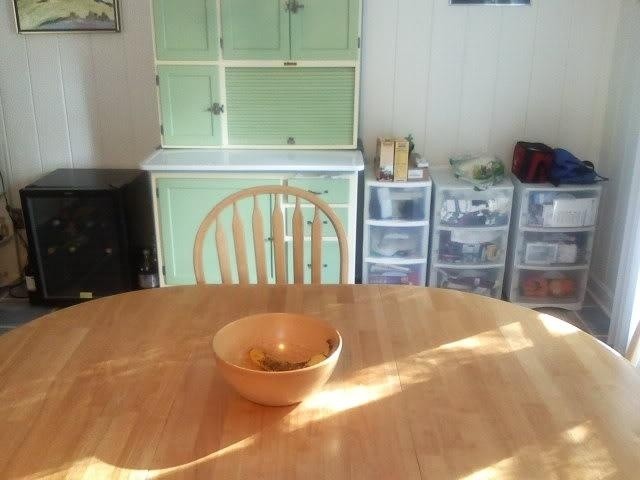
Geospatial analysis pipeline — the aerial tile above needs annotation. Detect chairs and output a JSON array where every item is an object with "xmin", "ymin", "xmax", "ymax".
[{"xmin": 192, "ymin": 184, "xmax": 350, "ymax": 286}]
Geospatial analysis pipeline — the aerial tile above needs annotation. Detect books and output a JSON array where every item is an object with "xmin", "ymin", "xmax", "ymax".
[{"xmin": 368, "ymin": 274, "xmax": 410, "ymax": 284}]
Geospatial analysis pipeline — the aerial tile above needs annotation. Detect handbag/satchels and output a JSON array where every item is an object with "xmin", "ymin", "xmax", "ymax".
[{"xmin": 512, "ymin": 141, "xmax": 606, "ymax": 187}]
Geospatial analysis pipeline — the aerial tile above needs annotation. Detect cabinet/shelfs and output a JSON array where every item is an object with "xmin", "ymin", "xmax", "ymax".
[
  {"xmin": 19, "ymin": 163, "xmax": 159, "ymax": 306},
  {"xmin": 140, "ymin": 146, "xmax": 369, "ymax": 289},
  {"xmin": 149, "ymin": 1, "xmax": 362, "ymax": 151}
]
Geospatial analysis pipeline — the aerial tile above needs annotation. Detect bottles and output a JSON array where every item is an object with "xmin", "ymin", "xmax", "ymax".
[
  {"xmin": 25, "ymin": 254, "xmax": 44, "ymax": 305},
  {"xmin": 43, "ymin": 209, "xmax": 115, "ymax": 258},
  {"xmin": 135, "ymin": 249, "xmax": 159, "ymax": 290}
]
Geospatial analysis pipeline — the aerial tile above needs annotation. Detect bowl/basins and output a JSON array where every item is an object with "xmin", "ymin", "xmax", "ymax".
[{"xmin": 209, "ymin": 314, "xmax": 343, "ymax": 411}]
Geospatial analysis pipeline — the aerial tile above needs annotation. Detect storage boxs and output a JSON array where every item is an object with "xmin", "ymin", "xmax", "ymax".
[
  {"xmin": 363, "ymin": 165, "xmax": 433, "ymax": 286},
  {"xmin": 428, "ymin": 164, "xmax": 515, "ymax": 300},
  {"xmin": 504, "ymin": 172, "xmax": 603, "ymax": 312}
]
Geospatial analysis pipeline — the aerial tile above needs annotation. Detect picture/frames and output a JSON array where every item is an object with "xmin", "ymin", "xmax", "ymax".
[
  {"xmin": 449, "ymin": 0, "xmax": 531, "ymax": 8},
  {"xmin": 11, "ymin": 1, "xmax": 122, "ymax": 35}
]
